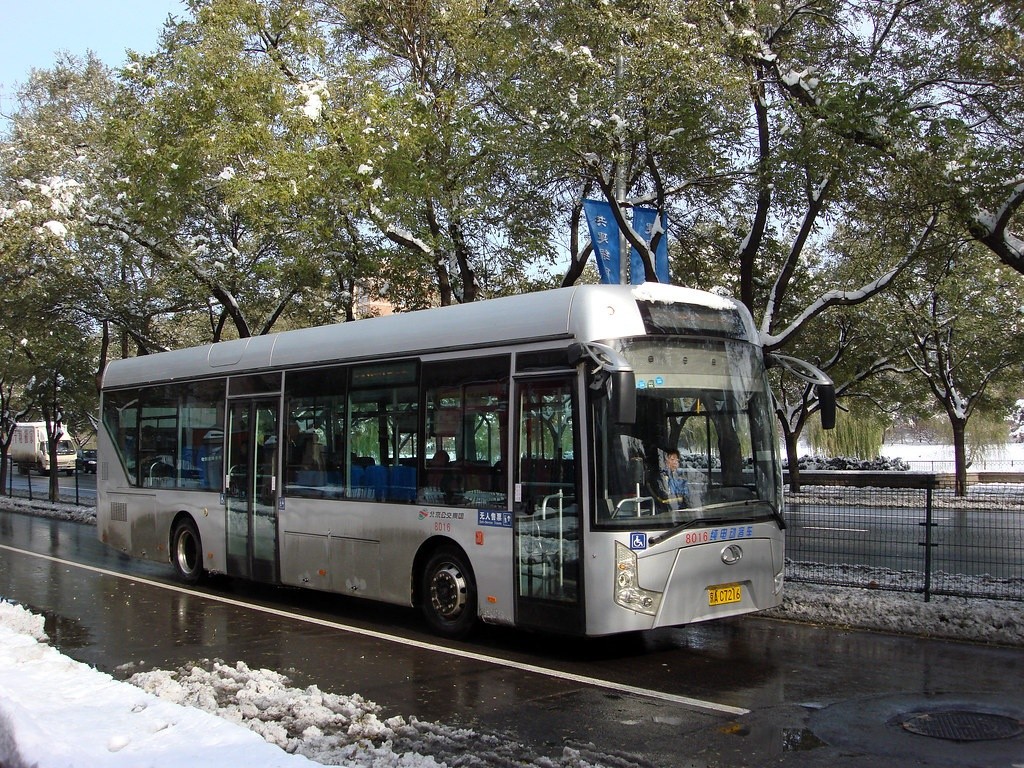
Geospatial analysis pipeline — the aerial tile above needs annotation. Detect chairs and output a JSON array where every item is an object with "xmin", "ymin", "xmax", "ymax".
[{"xmin": 124, "ymin": 431, "xmax": 575, "ymax": 509}]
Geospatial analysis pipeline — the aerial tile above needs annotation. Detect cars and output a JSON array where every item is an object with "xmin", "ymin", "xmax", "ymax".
[{"xmin": 76, "ymin": 449, "xmax": 97, "ymax": 474}]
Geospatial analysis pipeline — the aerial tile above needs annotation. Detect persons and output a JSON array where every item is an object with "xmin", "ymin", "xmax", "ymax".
[
  {"xmin": 426, "ymin": 450, "xmax": 451, "ymax": 487},
  {"xmin": 644, "ymin": 449, "xmax": 690, "ymax": 514},
  {"xmin": 125, "ymin": 425, "xmax": 162, "ymax": 478},
  {"xmin": 288, "ymin": 414, "xmax": 310, "ymax": 481}
]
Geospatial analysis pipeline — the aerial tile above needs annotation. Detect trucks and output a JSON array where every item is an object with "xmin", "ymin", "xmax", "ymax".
[{"xmin": 8, "ymin": 421, "xmax": 78, "ymax": 477}]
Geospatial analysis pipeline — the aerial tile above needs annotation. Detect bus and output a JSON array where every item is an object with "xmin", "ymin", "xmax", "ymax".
[{"xmin": 94, "ymin": 281, "xmax": 838, "ymax": 645}]
